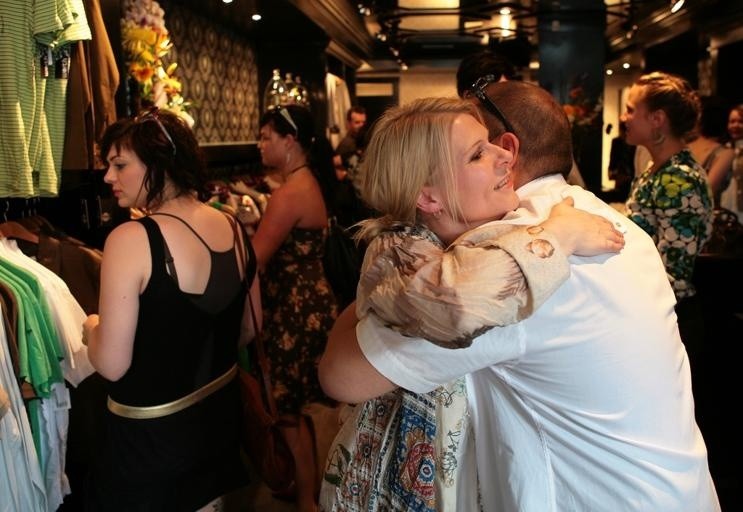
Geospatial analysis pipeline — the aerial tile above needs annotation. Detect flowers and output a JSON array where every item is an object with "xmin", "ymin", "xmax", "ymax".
[{"xmin": 118, "ymin": 0, "xmax": 195, "ymax": 131}]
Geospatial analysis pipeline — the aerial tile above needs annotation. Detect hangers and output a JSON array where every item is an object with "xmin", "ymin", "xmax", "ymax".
[{"xmin": 0, "ymin": 197, "xmax": 59, "ymax": 260}]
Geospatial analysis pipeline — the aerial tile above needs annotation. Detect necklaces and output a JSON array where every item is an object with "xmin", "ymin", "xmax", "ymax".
[{"xmin": 285, "ymin": 163, "xmax": 307, "ymax": 179}]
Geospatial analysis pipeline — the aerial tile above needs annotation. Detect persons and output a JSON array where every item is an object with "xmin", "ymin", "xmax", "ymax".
[
  {"xmin": 342, "ymin": 125, "xmax": 375, "ymax": 177},
  {"xmin": 80, "ymin": 107, "xmax": 267, "ymax": 511},
  {"xmin": 456, "ymin": 50, "xmax": 518, "ymax": 101},
  {"xmin": 318, "ymin": 98, "xmax": 628, "ymax": 511},
  {"xmin": 318, "ymin": 80, "xmax": 723, "ymax": 511},
  {"xmin": 616, "ymin": 72, "xmax": 717, "ymax": 394},
  {"xmin": 330, "ymin": 146, "xmax": 348, "ymax": 181},
  {"xmin": 246, "ymin": 105, "xmax": 338, "ymax": 511},
  {"xmin": 606, "ymin": 119, "xmax": 637, "ymax": 203},
  {"xmin": 684, "ymin": 88, "xmax": 736, "ymax": 209},
  {"xmin": 333, "ymin": 104, "xmax": 369, "ymax": 156},
  {"xmin": 720, "ymin": 103, "xmax": 743, "ymax": 227}
]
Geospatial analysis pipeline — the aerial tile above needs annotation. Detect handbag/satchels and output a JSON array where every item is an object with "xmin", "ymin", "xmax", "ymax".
[{"xmin": 235, "ymin": 334, "xmax": 281, "ymax": 430}]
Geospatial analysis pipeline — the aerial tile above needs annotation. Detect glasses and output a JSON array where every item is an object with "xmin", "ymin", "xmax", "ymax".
[
  {"xmin": 132, "ymin": 105, "xmax": 179, "ymax": 161},
  {"xmin": 464, "ymin": 75, "xmax": 514, "ymax": 135}
]
